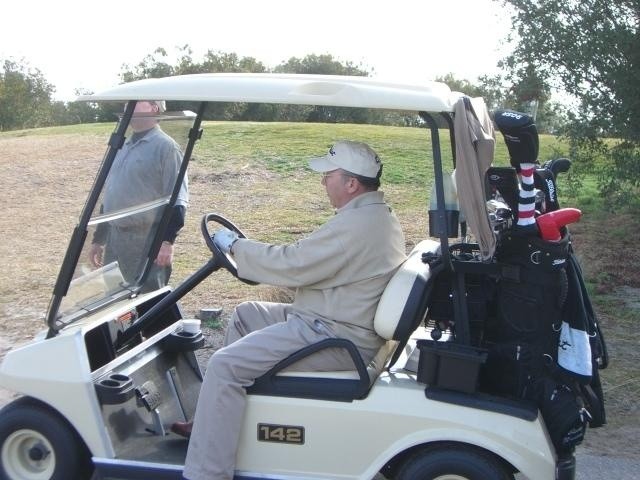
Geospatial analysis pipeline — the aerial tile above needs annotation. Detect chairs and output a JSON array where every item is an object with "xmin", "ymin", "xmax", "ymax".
[{"xmin": 240, "ymin": 239, "xmax": 442, "ymax": 400}]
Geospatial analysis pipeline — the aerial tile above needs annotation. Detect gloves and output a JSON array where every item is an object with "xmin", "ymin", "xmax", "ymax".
[{"xmin": 212, "ymin": 230, "xmax": 238, "ymax": 253}]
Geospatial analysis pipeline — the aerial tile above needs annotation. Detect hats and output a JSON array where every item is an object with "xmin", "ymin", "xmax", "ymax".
[
  {"xmin": 308, "ymin": 140, "xmax": 383, "ymax": 178},
  {"xmin": 153, "ymin": 101, "xmax": 167, "ymax": 114}
]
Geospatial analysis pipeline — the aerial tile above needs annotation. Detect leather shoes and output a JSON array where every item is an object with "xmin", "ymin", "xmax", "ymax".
[{"xmin": 170, "ymin": 420, "xmax": 194, "ymax": 437}]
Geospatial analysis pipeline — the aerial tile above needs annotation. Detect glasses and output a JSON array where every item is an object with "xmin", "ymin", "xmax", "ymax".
[{"xmin": 321, "ymin": 172, "xmax": 332, "ymax": 182}]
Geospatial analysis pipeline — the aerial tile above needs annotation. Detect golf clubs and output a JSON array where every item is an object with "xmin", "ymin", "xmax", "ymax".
[{"xmin": 486, "ymin": 109, "xmax": 581, "ymax": 242}]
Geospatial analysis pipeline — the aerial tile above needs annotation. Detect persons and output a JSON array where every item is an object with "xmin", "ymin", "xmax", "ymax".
[
  {"xmin": 86, "ymin": 101, "xmax": 190, "ymax": 292},
  {"xmin": 169, "ymin": 136, "xmax": 407, "ymax": 479}
]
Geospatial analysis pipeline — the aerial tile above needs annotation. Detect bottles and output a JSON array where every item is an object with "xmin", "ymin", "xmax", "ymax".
[{"xmin": 428, "ymin": 170, "xmax": 458, "ymax": 210}]
